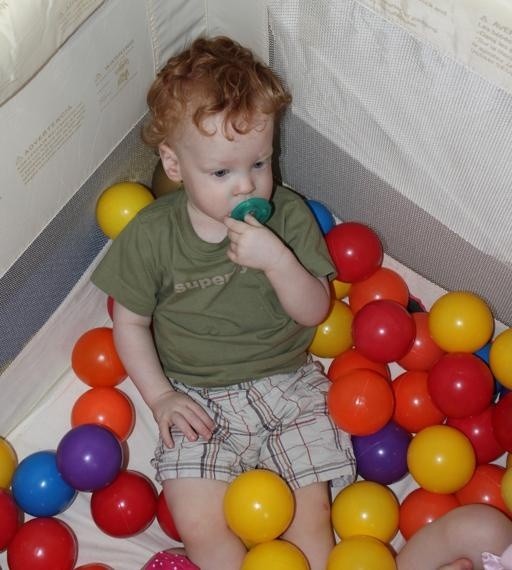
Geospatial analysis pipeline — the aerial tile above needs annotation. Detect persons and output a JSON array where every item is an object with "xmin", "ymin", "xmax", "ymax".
[
  {"xmin": 394, "ymin": 504, "xmax": 511, "ymax": 570},
  {"xmin": 87, "ymin": 33, "xmax": 354, "ymax": 570}
]
